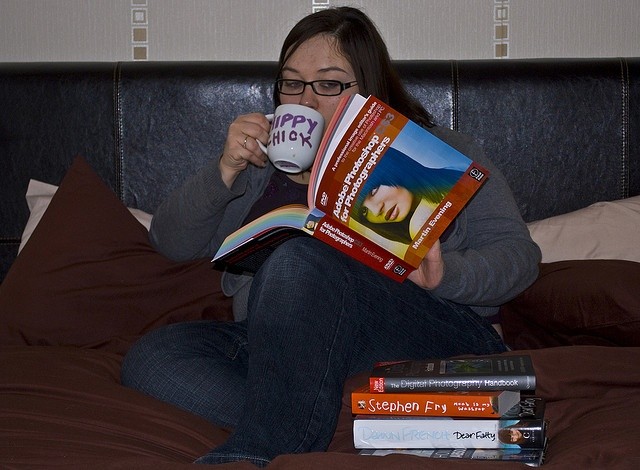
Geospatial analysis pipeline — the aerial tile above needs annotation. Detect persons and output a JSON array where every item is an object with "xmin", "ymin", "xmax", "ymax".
[
  {"xmin": 122, "ymin": 7, "xmax": 542, "ymax": 470},
  {"xmin": 498, "ymin": 418, "xmax": 542, "ymax": 453},
  {"xmin": 350, "ymin": 147, "xmax": 464, "ymax": 247}
]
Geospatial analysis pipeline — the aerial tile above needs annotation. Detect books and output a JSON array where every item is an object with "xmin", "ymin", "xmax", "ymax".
[
  {"xmin": 352, "ymin": 385, "xmax": 543, "ymax": 416},
  {"xmin": 358, "ymin": 435, "xmax": 555, "ymax": 466},
  {"xmin": 210, "ymin": 94, "xmax": 492, "ymax": 284},
  {"xmin": 352, "ymin": 395, "xmax": 551, "ymax": 449},
  {"xmin": 370, "ymin": 356, "xmax": 537, "ymax": 393}
]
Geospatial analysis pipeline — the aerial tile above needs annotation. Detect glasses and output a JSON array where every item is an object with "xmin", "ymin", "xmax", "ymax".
[{"xmin": 272, "ymin": 76, "xmax": 357, "ymax": 97}]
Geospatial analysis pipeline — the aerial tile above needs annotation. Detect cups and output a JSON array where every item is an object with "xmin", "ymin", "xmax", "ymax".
[{"xmin": 256, "ymin": 103, "xmax": 324, "ymax": 174}]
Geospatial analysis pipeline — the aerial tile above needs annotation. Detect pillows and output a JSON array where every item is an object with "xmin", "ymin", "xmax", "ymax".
[
  {"xmin": 20, "ymin": 178, "xmax": 154, "ymax": 234},
  {"xmin": 0, "ymin": 155, "xmax": 226, "ymax": 346},
  {"xmin": 520, "ymin": 193, "xmax": 639, "ymax": 264},
  {"xmin": 514, "ymin": 259, "xmax": 636, "ymax": 343}
]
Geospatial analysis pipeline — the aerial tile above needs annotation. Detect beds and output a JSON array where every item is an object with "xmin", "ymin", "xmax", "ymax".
[{"xmin": 0, "ymin": 61, "xmax": 639, "ymax": 468}]
